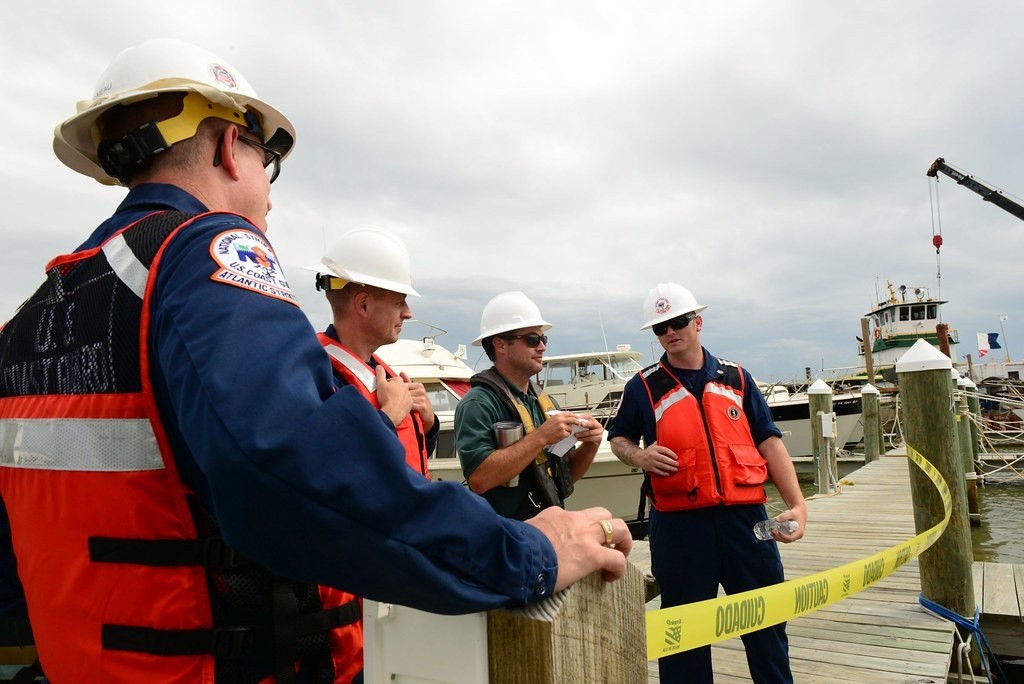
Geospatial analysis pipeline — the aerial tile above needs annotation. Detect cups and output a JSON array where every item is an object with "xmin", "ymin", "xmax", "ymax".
[{"xmin": 492, "ymin": 421, "xmax": 523, "ymax": 487}]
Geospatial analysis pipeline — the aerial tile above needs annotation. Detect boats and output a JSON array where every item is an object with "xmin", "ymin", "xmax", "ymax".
[
  {"xmin": 774, "ymin": 277, "xmax": 1024, "ymax": 395},
  {"xmin": 373, "ymin": 320, "xmax": 649, "ymax": 541},
  {"xmin": 751, "ymin": 376, "xmax": 893, "ymax": 453},
  {"xmin": 530, "ymin": 311, "xmax": 861, "ymax": 458}
]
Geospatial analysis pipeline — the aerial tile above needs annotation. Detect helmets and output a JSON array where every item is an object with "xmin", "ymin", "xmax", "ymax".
[
  {"xmin": 471, "ymin": 291, "xmax": 552, "ymax": 346},
  {"xmin": 52, "ymin": 38, "xmax": 296, "ymax": 187},
  {"xmin": 300, "ymin": 227, "xmax": 422, "ymax": 297},
  {"xmin": 640, "ymin": 282, "xmax": 708, "ymax": 330}
]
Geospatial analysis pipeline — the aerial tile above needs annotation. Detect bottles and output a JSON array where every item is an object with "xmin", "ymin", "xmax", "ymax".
[{"xmin": 753, "ymin": 520, "xmax": 799, "ymax": 540}]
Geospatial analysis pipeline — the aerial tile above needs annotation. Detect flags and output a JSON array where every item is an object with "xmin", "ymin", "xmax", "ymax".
[{"xmin": 977, "ymin": 332, "xmax": 1002, "ymax": 350}]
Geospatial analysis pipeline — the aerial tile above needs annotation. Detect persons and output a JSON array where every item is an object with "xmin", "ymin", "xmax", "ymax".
[
  {"xmin": 300, "ymin": 226, "xmax": 441, "ymax": 482},
  {"xmin": 607, "ymin": 281, "xmax": 808, "ymax": 684},
  {"xmin": 0, "ymin": 35, "xmax": 632, "ymax": 684},
  {"xmin": 454, "ymin": 292, "xmax": 603, "ymax": 521}
]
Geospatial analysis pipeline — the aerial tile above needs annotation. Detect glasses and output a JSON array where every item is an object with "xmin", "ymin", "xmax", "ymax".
[
  {"xmin": 652, "ymin": 314, "xmax": 694, "ymax": 336},
  {"xmin": 212, "ymin": 130, "xmax": 281, "ymax": 184},
  {"xmin": 500, "ymin": 332, "xmax": 548, "ymax": 348}
]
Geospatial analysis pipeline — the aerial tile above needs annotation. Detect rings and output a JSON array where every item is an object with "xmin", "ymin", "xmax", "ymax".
[{"xmin": 600, "ymin": 520, "xmax": 613, "ymax": 545}]
